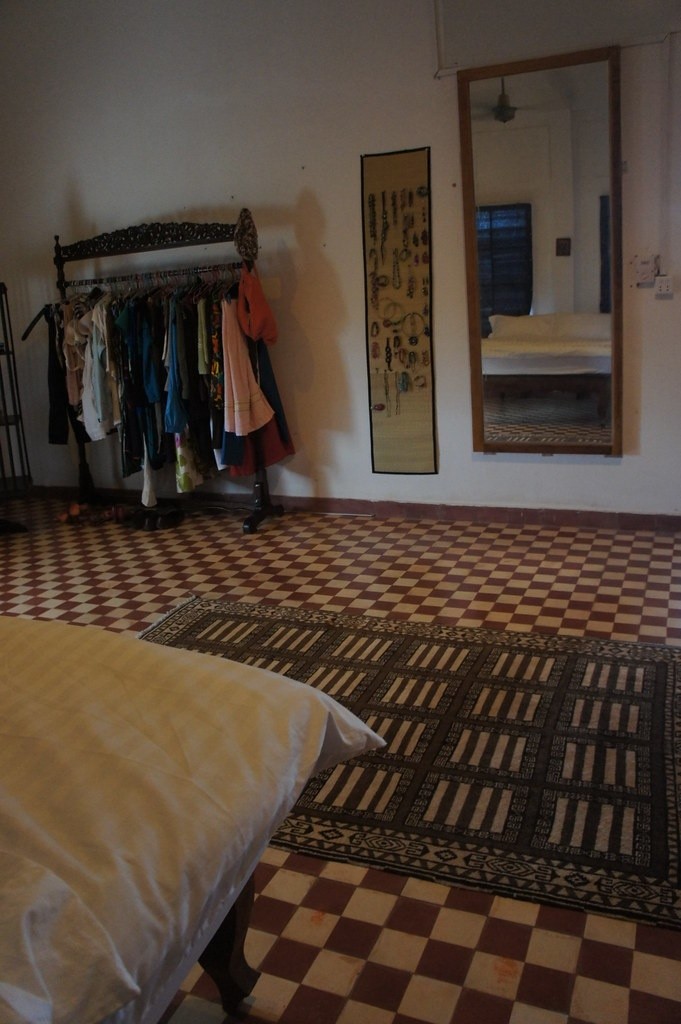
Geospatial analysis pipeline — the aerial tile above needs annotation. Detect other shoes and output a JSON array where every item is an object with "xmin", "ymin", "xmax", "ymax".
[
  {"xmin": 69, "ymin": 503, "xmax": 80, "ymax": 517},
  {"xmin": 144, "ymin": 510, "xmax": 161, "ymax": 530},
  {"xmin": 122, "ymin": 508, "xmax": 144, "ymax": 529},
  {"xmin": 157, "ymin": 508, "xmax": 182, "ymax": 528},
  {"xmin": 104, "ymin": 504, "xmax": 127, "ymax": 523},
  {"xmin": 57, "ymin": 510, "xmax": 69, "ymax": 522}
]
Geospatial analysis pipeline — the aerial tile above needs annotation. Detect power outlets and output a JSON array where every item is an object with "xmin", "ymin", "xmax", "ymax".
[{"xmin": 655, "ymin": 276, "xmax": 674, "ymax": 297}]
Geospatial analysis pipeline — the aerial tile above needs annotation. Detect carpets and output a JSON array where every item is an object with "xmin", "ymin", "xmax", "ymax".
[{"xmin": 133, "ymin": 594, "xmax": 681, "ymax": 929}]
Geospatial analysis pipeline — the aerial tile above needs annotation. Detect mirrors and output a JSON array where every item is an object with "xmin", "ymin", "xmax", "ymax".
[{"xmin": 457, "ymin": 44, "xmax": 622, "ymax": 457}]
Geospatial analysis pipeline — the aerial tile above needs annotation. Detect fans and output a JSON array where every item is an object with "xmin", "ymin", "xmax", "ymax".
[{"xmin": 470, "ymin": 77, "xmax": 572, "ymax": 123}]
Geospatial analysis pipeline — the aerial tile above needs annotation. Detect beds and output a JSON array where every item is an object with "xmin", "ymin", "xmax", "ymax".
[
  {"xmin": 481, "ymin": 313, "xmax": 611, "ymax": 392},
  {"xmin": 0, "ymin": 612, "xmax": 388, "ymax": 1024}
]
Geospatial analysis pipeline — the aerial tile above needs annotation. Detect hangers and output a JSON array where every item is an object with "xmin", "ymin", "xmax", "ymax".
[{"xmin": 50, "ymin": 261, "xmax": 245, "ymax": 313}]
